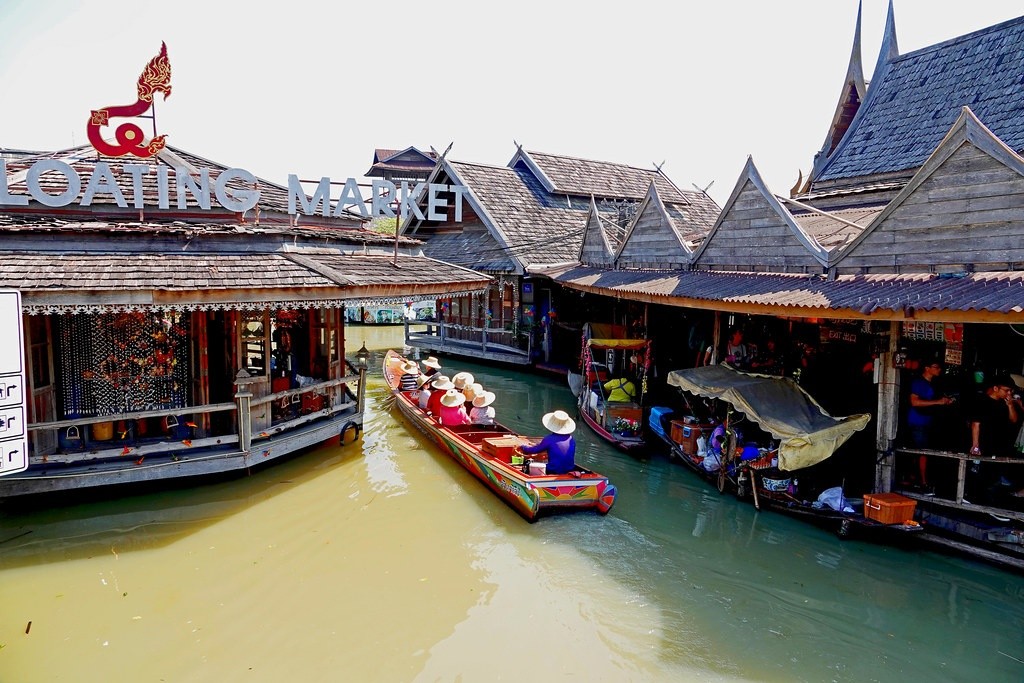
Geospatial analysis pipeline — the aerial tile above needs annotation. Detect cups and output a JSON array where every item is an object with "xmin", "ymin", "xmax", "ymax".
[{"xmin": 975, "ymin": 372, "xmax": 984, "ymax": 383}]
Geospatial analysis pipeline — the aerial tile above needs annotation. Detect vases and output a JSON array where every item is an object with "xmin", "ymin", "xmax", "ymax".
[{"xmin": 620, "ymin": 431, "xmax": 631, "ymax": 437}]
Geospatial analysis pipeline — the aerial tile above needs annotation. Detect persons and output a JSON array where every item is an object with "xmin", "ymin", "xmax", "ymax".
[
  {"xmin": 965, "ymin": 380, "xmax": 1024, "ymax": 532},
  {"xmin": 469, "ymin": 391, "xmax": 496, "ymax": 425},
  {"xmin": 416, "ymin": 373, "xmax": 482, "ymax": 417},
  {"xmin": 604, "ymin": 369, "xmax": 637, "ymax": 402},
  {"xmin": 694, "ymin": 326, "xmax": 785, "ymax": 377},
  {"xmin": 514, "ymin": 411, "xmax": 577, "ymax": 475},
  {"xmin": 439, "ymin": 390, "xmax": 471, "ymax": 426},
  {"xmin": 398, "ymin": 361, "xmax": 421, "ymax": 392},
  {"xmin": 706, "ymin": 418, "xmax": 734, "ymax": 453},
  {"xmin": 422, "ymin": 356, "xmax": 442, "ymax": 376},
  {"xmin": 901, "ymin": 358, "xmax": 954, "ymax": 495}
]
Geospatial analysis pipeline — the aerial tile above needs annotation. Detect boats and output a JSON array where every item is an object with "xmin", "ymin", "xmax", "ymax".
[
  {"xmin": 382, "ymin": 349, "xmax": 618, "ymax": 524},
  {"xmin": 568, "ymin": 323, "xmax": 652, "ymax": 450},
  {"xmin": 648, "ymin": 362, "xmax": 924, "ymax": 551}
]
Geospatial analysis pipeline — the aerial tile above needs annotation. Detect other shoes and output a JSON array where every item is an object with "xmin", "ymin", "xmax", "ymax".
[{"xmin": 1010, "ymin": 490, "xmax": 1023, "ymax": 497}]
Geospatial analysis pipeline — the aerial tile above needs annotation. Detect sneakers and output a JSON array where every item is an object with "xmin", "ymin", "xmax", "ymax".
[{"xmin": 920, "ymin": 481, "xmax": 935, "ymax": 497}]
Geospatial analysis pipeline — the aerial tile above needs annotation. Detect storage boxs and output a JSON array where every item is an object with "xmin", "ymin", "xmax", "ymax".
[
  {"xmin": 862, "ymin": 492, "xmax": 918, "ymax": 524},
  {"xmin": 601, "ymin": 401, "xmax": 642, "ymax": 430},
  {"xmin": 671, "ymin": 419, "xmax": 719, "ymax": 455}
]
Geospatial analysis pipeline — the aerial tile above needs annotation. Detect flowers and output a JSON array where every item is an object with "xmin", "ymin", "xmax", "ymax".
[{"xmin": 614, "ymin": 417, "xmax": 640, "ymax": 432}]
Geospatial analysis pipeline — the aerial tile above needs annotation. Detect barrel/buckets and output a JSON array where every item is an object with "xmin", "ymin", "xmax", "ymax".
[
  {"xmin": 92, "ymin": 422, "xmax": 113, "ymax": 440},
  {"xmin": 529, "ymin": 463, "xmax": 546, "ymax": 476}
]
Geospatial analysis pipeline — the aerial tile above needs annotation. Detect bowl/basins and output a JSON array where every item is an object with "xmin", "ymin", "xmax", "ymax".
[
  {"xmin": 683, "ymin": 416, "xmax": 695, "ymax": 424},
  {"xmin": 761, "ymin": 469, "xmax": 791, "ymax": 492},
  {"xmin": 511, "ymin": 455, "xmax": 525, "ymax": 464}
]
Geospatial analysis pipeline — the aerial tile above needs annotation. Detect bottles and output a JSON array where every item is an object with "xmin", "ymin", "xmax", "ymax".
[
  {"xmin": 971, "ymin": 447, "xmax": 981, "ymax": 472},
  {"xmin": 282, "ymin": 370, "xmax": 285, "ymax": 377},
  {"xmin": 439, "ymin": 417, "xmax": 442, "ymax": 425}
]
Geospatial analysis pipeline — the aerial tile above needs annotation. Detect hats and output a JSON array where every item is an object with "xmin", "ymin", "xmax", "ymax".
[
  {"xmin": 416, "ymin": 375, "xmax": 433, "ymax": 390},
  {"xmin": 462, "ymin": 383, "xmax": 483, "ymax": 402},
  {"xmin": 431, "ymin": 376, "xmax": 455, "ymax": 390},
  {"xmin": 472, "ymin": 391, "xmax": 496, "ymax": 408},
  {"xmin": 400, "ymin": 361, "xmax": 418, "ymax": 374},
  {"xmin": 453, "ymin": 372, "xmax": 474, "ymax": 390},
  {"xmin": 542, "ymin": 410, "xmax": 576, "ymax": 434},
  {"xmin": 440, "ymin": 389, "xmax": 466, "ymax": 407},
  {"xmin": 422, "ymin": 357, "xmax": 442, "ymax": 369}
]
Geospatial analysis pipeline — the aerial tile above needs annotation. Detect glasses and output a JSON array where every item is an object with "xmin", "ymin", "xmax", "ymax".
[
  {"xmin": 999, "ymin": 388, "xmax": 1013, "ymax": 393},
  {"xmin": 931, "ymin": 366, "xmax": 940, "ymax": 369}
]
